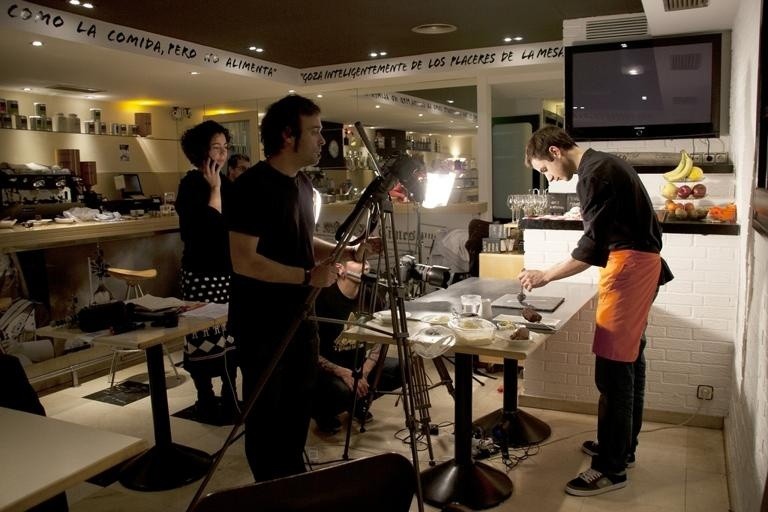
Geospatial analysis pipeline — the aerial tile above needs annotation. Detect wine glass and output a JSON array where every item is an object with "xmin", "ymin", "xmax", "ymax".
[{"xmin": 506, "ymin": 193, "xmax": 549, "ymax": 226}]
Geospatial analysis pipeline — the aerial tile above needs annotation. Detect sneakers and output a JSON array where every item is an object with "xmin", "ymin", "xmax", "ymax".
[
  {"xmin": 315, "ymin": 415, "xmax": 345, "ymax": 432},
  {"xmin": 581, "ymin": 439, "xmax": 637, "ymax": 468},
  {"xmin": 564, "ymin": 465, "xmax": 629, "ymax": 496},
  {"xmin": 349, "ymin": 405, "xmax": 374, "ymax": 425}
]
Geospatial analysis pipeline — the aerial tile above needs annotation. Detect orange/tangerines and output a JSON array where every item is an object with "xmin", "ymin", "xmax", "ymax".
[{"xmin": 665, "ymin": 198, "xmax": 695, "ymax": 211}]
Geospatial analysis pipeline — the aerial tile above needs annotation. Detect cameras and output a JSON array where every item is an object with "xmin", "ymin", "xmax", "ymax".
[
  {"xmin": 386, "ymin": 149, "xmax": 428, "ymax": 203},
  {"xmin": 394, "ymin": 254, "xmax": 451, "ymax": 290}
]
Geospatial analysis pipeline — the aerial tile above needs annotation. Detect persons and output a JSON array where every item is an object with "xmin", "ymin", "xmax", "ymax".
[
  {"xmin": 515, "ymin": 123, "xmax": 677, "ymax": 498},
  {"xmin": 222, "ymin": 92, "xmax": 385, "ymax": 484},
  {"xmin": 174, "ymin": 118, "xmax": 239, "ymax": 417},
  {"xmin": 225, "ymin": 152, "xmax": 251, "ymax": 181},
  {"xmin": 310, "ymin": 258, "xmax": 412, "ymax": 434}
]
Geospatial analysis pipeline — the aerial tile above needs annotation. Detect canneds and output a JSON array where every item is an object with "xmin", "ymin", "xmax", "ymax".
[
  {"xmin": 0, "ymin": 98, "xmax": 27, "ymax": 129},
  {"xmin": 85, "ymin": 108, "xmax": 107, "ymax": 135},
  {"xmin": 112, "ymin": 123, "xmax": 138, "ymax": 138},
  {"xmin": 29, "ymin": 103, "xmax": 52, "ymax": 132}
]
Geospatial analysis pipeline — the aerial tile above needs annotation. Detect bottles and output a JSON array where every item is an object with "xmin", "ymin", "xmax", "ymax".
[
  {"xmin": 405, "ymin": 134, "xmax": 441, "ymax": 153},
  {"xmin": 51, "ymin": 111, "xmax": 81, "ymax": 132}
]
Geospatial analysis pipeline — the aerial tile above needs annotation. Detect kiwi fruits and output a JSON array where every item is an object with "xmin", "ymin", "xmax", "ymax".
[{"xmin": 674, "ymin": 206, "xmax": 706, "ymax": 219}]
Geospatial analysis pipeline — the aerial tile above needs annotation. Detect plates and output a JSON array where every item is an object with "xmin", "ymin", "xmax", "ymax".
[
  {"xmin": 372, "ymin": 309, "xmax": 412, "ymax": 324},
  {"xmin": 492, "ymin": 313, "xmax": 562, "ymax": 331},
  {"xmin": 489, "ymin": 292, "xmax": 566, "ymax": 313}
]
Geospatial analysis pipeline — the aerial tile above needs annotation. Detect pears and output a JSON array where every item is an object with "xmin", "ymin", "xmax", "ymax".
[{"xmin": 662, "ymin": 184, "xmax": 678, "ymax": 199}]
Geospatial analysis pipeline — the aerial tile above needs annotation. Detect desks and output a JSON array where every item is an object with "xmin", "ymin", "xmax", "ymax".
[
  {"xmin": 36, "ymin": 296, "xmax": 230, "ymax": 494},
  {"xmin": 341, "ymin": 273, "xmax": 602, "ymax": 507}
]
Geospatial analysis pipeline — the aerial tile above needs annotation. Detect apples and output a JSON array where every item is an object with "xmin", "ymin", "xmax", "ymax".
[{"xmin": 678, "ymin": 184, "xmax": 706, "ymax": 198}]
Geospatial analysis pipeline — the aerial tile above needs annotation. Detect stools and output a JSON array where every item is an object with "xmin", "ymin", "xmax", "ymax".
[{"xmin": 107, "ymin": 265, "xmax": 184, "ymax": 389}]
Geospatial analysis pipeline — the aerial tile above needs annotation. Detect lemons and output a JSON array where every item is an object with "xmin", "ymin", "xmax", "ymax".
[{"xmin": 690, "ymin": 166, "xmax": 704, "ymax": 182}]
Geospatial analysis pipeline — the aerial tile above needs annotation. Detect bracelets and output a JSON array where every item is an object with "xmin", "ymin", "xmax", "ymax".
[
  {"xmin": 350, "ymin": 246, "xmax": 356, "ymax": 263},
  {"xmin": 302, "ymin": 269, "xmax": 311, "ymax": 286}
]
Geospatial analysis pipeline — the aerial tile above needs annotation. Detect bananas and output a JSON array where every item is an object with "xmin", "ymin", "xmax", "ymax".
[{"xmin": 663, "ymin": 150, "xmax": 693, "ymax": 182}]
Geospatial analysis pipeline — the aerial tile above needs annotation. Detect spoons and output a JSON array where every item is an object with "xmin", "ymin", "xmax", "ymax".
[{"xmin": 516, "ymin": 266, "xmax": 530, "ymax": 302}]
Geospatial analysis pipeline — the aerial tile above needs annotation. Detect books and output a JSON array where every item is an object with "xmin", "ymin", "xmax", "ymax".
[{"xmin": 123, "ymin": 294, "xmax": 184, "ymax": 312}]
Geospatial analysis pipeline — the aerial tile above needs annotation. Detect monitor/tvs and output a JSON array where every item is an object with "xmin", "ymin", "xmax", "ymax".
[{"xmin": 564, "ymin": 32, "xmax": 722, "ymax": 142}]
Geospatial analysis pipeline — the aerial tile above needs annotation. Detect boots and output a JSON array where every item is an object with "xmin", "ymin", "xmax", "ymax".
[
  {"xmin": 189, "ymin": 376, "xmax": 219, "ymax": 412},
  {"xmin": 220, "ymin": 378, "xmax": 238, "ymax": 408}
]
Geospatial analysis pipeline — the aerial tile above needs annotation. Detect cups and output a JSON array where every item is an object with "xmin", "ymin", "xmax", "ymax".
[
  {"xmin": 129, "ymin": 209, "xmax": 145, "ymax": 217},
  {"xmin": 460, "ymin": 293, "xmax": 483, "ymax": 315},
  {"xmin": 159, "ymin": 204, "xmax": 172, "ymax": 217}
]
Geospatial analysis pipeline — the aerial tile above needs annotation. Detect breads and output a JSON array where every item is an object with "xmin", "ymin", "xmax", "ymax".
[
  {"xmin": 522, "ymin": 306, "xmax": 542, "ymax": 323},
  {"xmin": 510, "ymin": 326, "xmax": 531, "ymax": 340}
]
